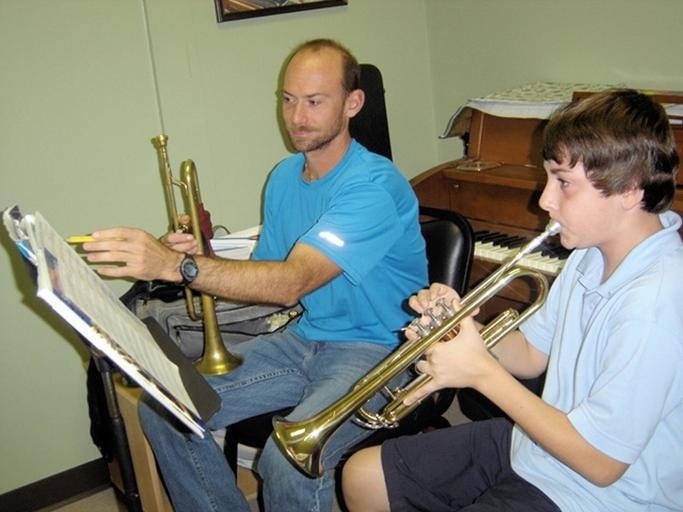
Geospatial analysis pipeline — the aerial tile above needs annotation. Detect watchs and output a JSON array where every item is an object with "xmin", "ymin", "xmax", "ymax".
[{"xmin": 175, "ymin": 252, "xmax": 198, "ymax": 288}]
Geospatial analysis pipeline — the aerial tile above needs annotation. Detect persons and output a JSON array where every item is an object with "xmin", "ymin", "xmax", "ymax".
[
  {"xmin": 82, "ymin": 38, "xmax": 430, "ymax": 512},
  {"xmin": 340, "ymin": 88, "xmax": 683, "ymax": 512}
]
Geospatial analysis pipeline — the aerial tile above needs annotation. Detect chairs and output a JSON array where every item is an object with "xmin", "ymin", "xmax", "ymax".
[{"xmin": 221, "ymin": 206, "xmax": 475, "ymax": 512}]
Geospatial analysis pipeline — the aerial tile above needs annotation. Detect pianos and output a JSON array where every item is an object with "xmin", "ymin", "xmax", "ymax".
[{"xmin": 409, "ymin": 83, "xmax": 683, "ymax": 423}]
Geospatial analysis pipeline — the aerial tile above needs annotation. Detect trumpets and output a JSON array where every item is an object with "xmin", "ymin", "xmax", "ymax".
[
  {"xmin": 151, "ymin": 135, "xmax": 243, "ymax": 376},
  {"xmin": 271, "ymin": 218, "xmax": 561, "ymax": 478}
]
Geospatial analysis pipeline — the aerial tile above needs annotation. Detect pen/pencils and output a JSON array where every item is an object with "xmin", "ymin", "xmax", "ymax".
[{"xmin": 65, "ymin": 236, "xmax": 94, "ymax": 243}]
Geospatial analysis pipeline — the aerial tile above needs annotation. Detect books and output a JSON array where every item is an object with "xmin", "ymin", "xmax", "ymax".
[{"xmin": 1, "ymin": 203, "xmax": 205, "ymax": 440}]
